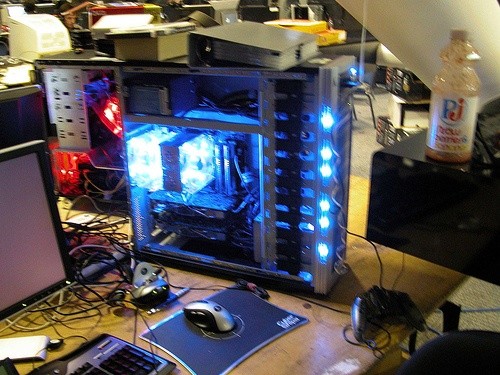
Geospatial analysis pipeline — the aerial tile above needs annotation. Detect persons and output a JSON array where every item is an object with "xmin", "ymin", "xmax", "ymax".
[{"xmin": 59, "ymin": 4, "xmax": 77, "ymax": 29}]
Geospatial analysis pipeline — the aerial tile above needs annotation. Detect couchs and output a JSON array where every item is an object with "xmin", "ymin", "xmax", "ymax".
[{"xmin": 317, "ymin": 41, "xmax": 398, "ymax": 84}]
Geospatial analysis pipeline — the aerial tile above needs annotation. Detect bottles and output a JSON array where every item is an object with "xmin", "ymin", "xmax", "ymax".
[{"xmin": 424, "ymin": 27, "xmax": 480, "ymax": 163}]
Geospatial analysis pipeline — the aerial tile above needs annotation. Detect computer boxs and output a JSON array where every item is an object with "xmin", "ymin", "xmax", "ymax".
[
  {"xmin": 113, "ymin": 52, "xmax": 357, "ymax": 302},
  {"xmin": 34, "ymin": 48, "xmax": 126, "ymax": 209}
]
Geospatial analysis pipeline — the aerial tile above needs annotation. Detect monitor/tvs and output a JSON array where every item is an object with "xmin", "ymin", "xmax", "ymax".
[
  {"xmin": 0, "ymin": 85, "xmax": 47, "ymax": 149},
  {"xmin": 0, "ymin": 140, "xmax": 75, "ymax": 320}
]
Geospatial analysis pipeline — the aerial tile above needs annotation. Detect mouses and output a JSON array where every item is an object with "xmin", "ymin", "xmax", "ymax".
[
  {"xmin": 130, "ymin": 278, "xmax": 169, "ymax": 308},
  {"xmin": 182, "ymin": 300, "xmax": 237, "ymax": 333}
]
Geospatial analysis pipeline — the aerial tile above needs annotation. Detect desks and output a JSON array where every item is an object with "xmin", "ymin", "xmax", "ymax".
[
  {"xmin": 1, "ymin": 199, "xmax": 465, "ymax": 374},
  {"xmin": 394, "ymin": 94, "xmax": 431, "ymax": 127},
  {"xmin": 0, "ymin": 47, "xmax": 110, "ymax": 89},
  {"xmin": 365, "ymin": 126, "xmax": 500, "ymax": 282}
]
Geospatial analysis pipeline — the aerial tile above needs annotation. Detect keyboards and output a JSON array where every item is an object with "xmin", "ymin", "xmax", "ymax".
[{"xmin": 22, "ymin": 334, "xmax": 176, "ymax": 375}]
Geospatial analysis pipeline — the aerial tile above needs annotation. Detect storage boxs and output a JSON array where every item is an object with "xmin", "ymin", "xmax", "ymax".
[
  {"xmin": 266, "ymin": 19, "xmax": 348, "ymax": 45},
  {"xmin": 105, "ymin": 21, "xmax": 198, "ymax": 62}
]
